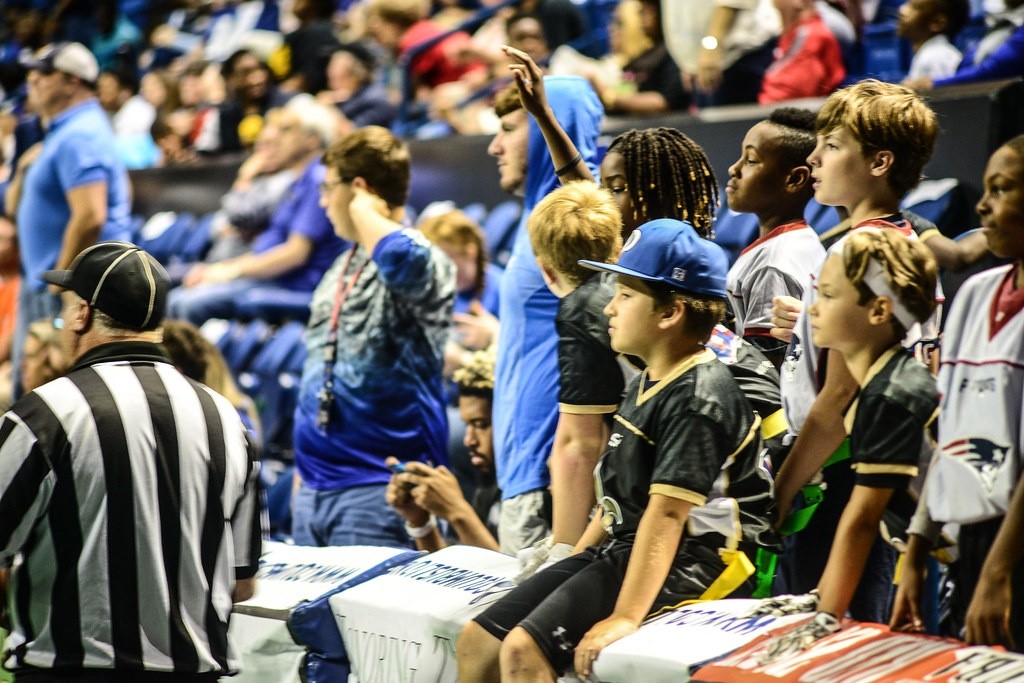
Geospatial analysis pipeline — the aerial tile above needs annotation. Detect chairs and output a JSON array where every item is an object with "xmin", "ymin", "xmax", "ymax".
[{"xmin": 127, "ymin": 176, "xmax": 983, "ymax": 456}]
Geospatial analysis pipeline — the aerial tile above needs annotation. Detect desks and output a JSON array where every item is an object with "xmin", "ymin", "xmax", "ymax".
[{"xmin": 217, "ymin": 540, "xmax": 1024, "ymax": 683}]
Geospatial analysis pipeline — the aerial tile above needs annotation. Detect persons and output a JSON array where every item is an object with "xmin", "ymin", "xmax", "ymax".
[{"xmin": 0, "ymin": 0, "xmax": 1024, "ymax": 683}]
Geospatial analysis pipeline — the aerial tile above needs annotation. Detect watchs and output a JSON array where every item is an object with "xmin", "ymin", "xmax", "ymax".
[{"xmin": 701, "ymin": 36, "xmax": 723, "ymax": 51}]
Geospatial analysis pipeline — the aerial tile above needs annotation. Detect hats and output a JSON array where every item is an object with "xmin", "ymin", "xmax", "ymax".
[
  {"xmin": 40, "ymin": 239, "xmax": 170, "ymax": 332},
  {"xmin": 17, "ymin": 41, "xmax": 100, "ymax": 88},
  {"xmin": 573, "ymin": 217, "xmax": 731, "ymax": 300},
  {"xmin": 105, "ymin": 43, "xmax": 138, "ymax": 87}
]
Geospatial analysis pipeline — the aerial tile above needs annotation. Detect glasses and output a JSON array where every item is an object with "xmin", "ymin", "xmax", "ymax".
[{"xmin": 319, "ymin": 179, "xmax": 355, "ymax": 196}]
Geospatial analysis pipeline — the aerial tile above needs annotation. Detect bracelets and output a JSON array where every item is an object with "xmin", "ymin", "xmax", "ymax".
[
  {"xmin": 405, "ymin": 515, "xmax": 437, "ymax": 538},
  {"xmin": 555, "ymin": 151, "xmax": 583, "ymax": 178}
]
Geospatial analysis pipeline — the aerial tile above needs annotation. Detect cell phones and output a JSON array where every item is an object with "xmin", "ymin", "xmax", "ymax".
[{"xmin": 390, "ymin": 462, "xmax": 422, "ymax": 490}]
